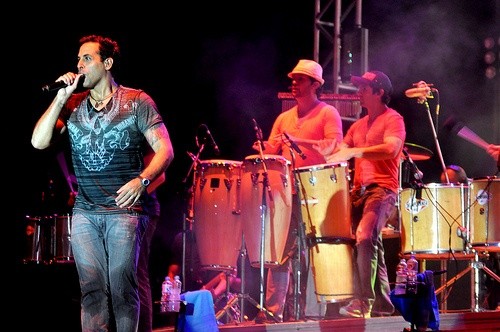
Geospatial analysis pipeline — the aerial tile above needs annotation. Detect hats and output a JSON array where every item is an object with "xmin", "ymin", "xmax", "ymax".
[
  {"xmin": 287, "ymin": 59, "xmax": 325, "ymax": 86},
  {"xmin": 351, "ymin": 71, "xmax": 393, "ymax": 96}
]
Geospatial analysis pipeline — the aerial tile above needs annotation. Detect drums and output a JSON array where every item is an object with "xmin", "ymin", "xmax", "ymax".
[
  {"xmin": 397, "ymin": 183, "xmax": 469, "ymax": 259},
  {"xmin": 469, "ymin": 176, "xmax": 500, "ymax": 249},
  {"xmin": 241, "ymin": 155, "xmax": 293, "ymax": 267},
  {"xmin": 192, "ymin": 158, "xmax": 243, "ymax": 273},
  {"xmin": 294, "ymin": 160, "xmax": 359, "ymax": 303},
  {"xmin": 22, "ymin": 215, "xmax": 73, "ymax": 265}
]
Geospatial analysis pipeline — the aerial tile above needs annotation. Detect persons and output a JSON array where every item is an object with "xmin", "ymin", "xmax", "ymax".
[
  {"xmin": 440, "ymin": 144, "xmax": 500, "ymax": 313},
  {"xmin": 252, "ymin": 59, "xmax": 344, "ymax": 323},
  {"xmin": 315, "ymin": 70, "xmax": 406, "ymax": 318},
  {"xmin": 30, "ymin": 35, "xmax": 174, "ymax": 332}
]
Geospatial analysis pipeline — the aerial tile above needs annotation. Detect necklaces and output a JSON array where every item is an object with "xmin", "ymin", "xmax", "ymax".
[{"xmin": 89, "ymin": 84, "xmax": 120, "ymax": 108}]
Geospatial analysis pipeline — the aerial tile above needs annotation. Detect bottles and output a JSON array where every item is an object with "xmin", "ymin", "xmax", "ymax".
[
  {"xmin": 407, "ymin": 254, "xmax": 418, "ymax": 295},
  {"xmin": 159, "ymin": 277, "xmax": 172, "ymax": 312},
  {"xmin": 395, "ymin": 259, "xmax": 407, "ymax": 294},
  {"xmin": 171, "ymin": 276, "xmax": 182, "ymax": 312}
]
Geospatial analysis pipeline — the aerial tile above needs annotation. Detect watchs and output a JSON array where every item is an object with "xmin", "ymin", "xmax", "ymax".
[{"xmin": 137, "ymin": 175, "xmax": 150, "ymax": 187}]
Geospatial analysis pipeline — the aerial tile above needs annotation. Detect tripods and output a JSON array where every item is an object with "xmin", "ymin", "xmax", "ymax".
[
  {"xmin": 215, "ymin": 232, "xmax": 280, "ymax": 324},
  {"xmin": 435, "ymin": 249, "xmax": 500, "ymax": 312}
]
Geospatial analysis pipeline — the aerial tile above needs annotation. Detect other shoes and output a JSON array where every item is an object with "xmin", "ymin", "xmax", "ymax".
[
  {"xmin": 339, "ymin": 298, "xmax": 372, "ymax": 319},
  {"xmin": 254, "ymin": 311, "xmax": 284, "ymax": 323}
]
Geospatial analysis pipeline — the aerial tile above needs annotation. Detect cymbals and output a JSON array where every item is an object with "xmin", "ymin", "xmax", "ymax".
[{"xmin": 400, "ymin": 143, "xmax": 433, "ymax": 160}]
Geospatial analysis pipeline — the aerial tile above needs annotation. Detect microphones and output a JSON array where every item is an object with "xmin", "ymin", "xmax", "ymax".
[
  {"xmin": 283, "ymin": 133, "xmax": 307, "ymax": 160},
  {"xmin": 252, "ymin": 119, "xmax": 265, "ymax": 151},
  {"xmin": 404, "ymin": 87, "xmax": 438, "ymax": 98},
  {"xmin": 41, "ymin": 74, "xmax": 77, "ymax": 92},
  {"xmin": 403, "ymin": 149, "xmax": 423, "ymax": 180},
  {"xmin": 199, "ymin": 124, "xmax": 220, "ymax": 155}
]
